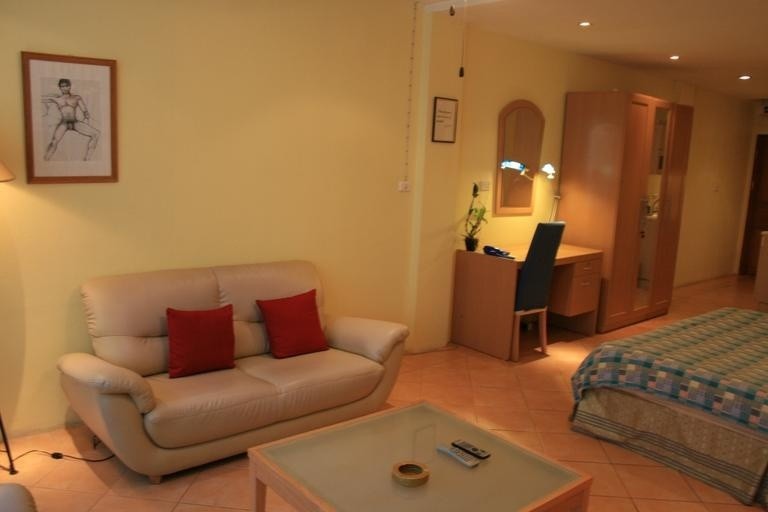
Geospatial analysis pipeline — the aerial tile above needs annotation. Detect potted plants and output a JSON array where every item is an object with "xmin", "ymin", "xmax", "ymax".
[{"xmin": 461, "ymin": 176, "xmax": 489, "ymax": 251}]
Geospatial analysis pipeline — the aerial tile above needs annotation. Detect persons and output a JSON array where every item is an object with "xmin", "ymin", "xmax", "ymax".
[{"xmin": 41, "ymin": 78, "xmax": 102, "ymax": 162}]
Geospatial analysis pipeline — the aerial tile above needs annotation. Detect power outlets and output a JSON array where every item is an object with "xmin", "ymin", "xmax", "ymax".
[{"xmin": 398, "ymin": 181, "xmax": 410, "ymax": 192}]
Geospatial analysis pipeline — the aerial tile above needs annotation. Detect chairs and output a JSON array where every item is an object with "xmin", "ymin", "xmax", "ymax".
[{"xmin": 513, "ymin": 223, "xmax": 566, "ymax": 363}]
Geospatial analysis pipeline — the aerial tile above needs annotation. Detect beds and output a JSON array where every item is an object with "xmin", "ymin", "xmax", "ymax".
[{"xmin": 569, "ymin": 303, "xmax": 768, "ymax": 512}]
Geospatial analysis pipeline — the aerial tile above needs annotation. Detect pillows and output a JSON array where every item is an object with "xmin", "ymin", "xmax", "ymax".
[
  {"xmin": 255, "ymin": 288, "xmax": 330, "ymax": 358},
  {"xmin": 158, "ymin": 302, "xmax": 241, "ymax": 379}
]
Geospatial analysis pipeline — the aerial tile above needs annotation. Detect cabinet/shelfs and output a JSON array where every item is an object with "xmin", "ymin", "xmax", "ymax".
[{"xmin": 561, "ymin": 91, "xmax": 695, "ymax": 333}]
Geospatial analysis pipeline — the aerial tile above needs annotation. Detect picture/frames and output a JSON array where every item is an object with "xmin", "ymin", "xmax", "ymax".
[
  {"xmin": 19, "ymin": 52, "xmax": 118, "ymax": 182},
  {"xmin": 432, "ymin": 97, "xmax": 460, "ymax": 143}
]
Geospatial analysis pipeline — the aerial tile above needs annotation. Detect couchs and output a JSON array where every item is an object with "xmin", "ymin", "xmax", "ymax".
[
  {"xmin": 54, "ymin": 260, "xmax": 411, "ymax": 485},
  {"xmin": 1, "ymin": 482, "xmax": 37, "ymax": 512}
]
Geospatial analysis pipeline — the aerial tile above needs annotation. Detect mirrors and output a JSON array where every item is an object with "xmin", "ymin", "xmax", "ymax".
[
  {"xmin": 634, "ymin": 106, "xmax": 669, "ymax": 310},
  {"xmin": 495, "ymin": 100, "xmax": 545, "ymax": 217}
]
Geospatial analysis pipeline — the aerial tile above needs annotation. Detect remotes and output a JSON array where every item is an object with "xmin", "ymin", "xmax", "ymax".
[
  {"xmin": 451, "ymin": 438, "xmax": 490, "ymax": 459},
  {"xmin": 436, "ymin": 444, "xmax": 480, "ymax": 468}
]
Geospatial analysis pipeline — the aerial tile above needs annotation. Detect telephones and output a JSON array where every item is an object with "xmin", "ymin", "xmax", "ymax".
[{"xmin": 484, "ymin": 245, "xmax": 511, "ymax": 257}]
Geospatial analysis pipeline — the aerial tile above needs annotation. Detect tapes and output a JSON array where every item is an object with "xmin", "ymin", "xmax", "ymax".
[{"xmin": 392, "ymin": 460, "xmax": 431, "ymax": 487}]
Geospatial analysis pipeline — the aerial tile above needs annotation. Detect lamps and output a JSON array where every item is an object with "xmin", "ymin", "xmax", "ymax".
[
  {"xmin": 501, "ymin": 161, "xmax": 534, "ymax": 183},
  {"xmin": 542, "ymin": 163, "xmax": 561, "ymax": 222}
]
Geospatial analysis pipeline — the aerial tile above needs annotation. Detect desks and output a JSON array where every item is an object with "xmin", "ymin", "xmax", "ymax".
[{"xmin": 454, "ymin": 242, "xmax": 603, "ymax": 361}]
